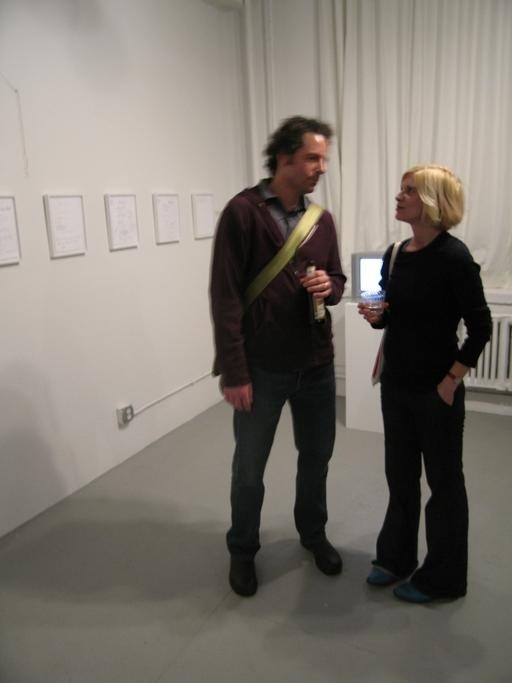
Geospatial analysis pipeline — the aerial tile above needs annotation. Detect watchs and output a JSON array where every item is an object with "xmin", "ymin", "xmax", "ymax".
[{"xmin": 447, "ymin": 370, "xmax": 463, "ymax": 385}]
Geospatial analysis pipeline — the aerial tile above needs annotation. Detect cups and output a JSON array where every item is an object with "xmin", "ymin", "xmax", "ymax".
[{"xmin": 359, "ymin": 288, "xmax": 386, "ymax": 316}]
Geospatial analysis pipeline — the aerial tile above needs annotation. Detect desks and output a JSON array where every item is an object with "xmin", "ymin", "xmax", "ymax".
[{"xmin": 344, "ymin": 303, "xmax": 384, "ymax": 432}]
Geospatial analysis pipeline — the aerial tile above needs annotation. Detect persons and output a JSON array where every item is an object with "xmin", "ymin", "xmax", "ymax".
[
  {"xmin": 210, "ymin": 117, "xmax": 347, "ymax": 596},
  {"xmin": 358, "ymin": 165, "xmax": 494, "ymax": 603}
]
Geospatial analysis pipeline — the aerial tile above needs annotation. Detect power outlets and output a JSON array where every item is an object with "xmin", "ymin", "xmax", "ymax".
[{"xmin": 118, "ymin": 406, "xmax": 134, "ymax": 427}]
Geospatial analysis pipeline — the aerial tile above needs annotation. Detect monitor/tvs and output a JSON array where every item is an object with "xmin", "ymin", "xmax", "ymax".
[{"xmin": 350, "ymin": 251, "xmax": 386, "ymax": 303}]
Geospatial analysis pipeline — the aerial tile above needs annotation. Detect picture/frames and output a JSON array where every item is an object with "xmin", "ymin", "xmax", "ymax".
[
  {"xmin": 0, "ymin": 196, "xmax": 22, "ymax": 267},
  {"xmin": 43, "ymin": 193, "xmax": 87, "ymax": 259},
  {"xmin": 192, "ymin": 194, "xmax": 216, "ymax": 240},
  {"xmin": 105, "ymin": 194, "xmax": 139, "ymax": 252},
  {"xmin": 153, "ymin": 193, "xmax": 179, "ymax": 245}
]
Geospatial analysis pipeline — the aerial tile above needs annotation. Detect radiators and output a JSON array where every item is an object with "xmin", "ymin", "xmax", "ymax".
[{"xmin": 462, "ymin": 314, "xmax": 512, "ymax": 392}]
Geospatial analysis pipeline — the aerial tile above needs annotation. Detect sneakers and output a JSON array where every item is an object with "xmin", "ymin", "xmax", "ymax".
[
  {"xmin": 296, "ymin": 524, "xmax": 347, "ymax": 579},
  {"xmin": 226, "ymin": 542, "xmax": 263, "ymax": 598},
  {"xmin": 389, "ymin": 577, "xmax": 471, "ymax": 606},
  {"xmin": 364, "ymin": 553, "xmax": 421, "ymax": 588}
]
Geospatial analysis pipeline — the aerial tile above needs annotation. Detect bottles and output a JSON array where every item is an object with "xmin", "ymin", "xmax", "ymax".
[{"xmin": 303, "ymin": 259, "xmax": 327, "ymax": 326}]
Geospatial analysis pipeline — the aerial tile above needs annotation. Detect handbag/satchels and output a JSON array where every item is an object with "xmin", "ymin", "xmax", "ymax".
[{"xmin": 370, "ymin": 327, "xmax": 390, "ymax": 390}]
